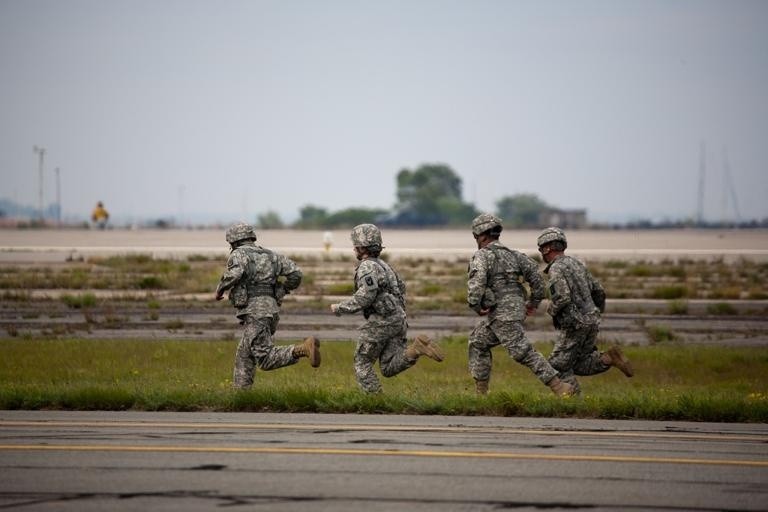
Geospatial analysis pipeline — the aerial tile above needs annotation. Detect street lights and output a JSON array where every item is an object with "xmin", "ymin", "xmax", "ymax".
[
  {"xmin": 33, "ymin": 146, "xmax": 46, "ymax": 219},
  {"xmin": 51, "ymin": 165, "xmax": 63, "ymax": 225}
]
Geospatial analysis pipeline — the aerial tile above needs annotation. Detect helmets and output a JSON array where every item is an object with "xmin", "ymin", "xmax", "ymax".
[
  {"xmin": 533, "ymin": 225, "xmax": 569, "ymax": 248},
  {"xmin": 469, "ymin": 212, "xmax": 505, "ymax": 237},
  {"xmin": 224, "ymin": 221, "xmax": 258, "ymax": 244},
  {"xmin": 350, "ymin": 222, "xmax": 383, "ymax": 249}
]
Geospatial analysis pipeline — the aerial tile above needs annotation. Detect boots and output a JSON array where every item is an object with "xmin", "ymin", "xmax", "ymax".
[
  {"xmin": 408, "ymin": 333, "xmax": 447, "ymax": 364},
  {"xmin": 600, "ymin": 342, "xmax": 636, "ymax": 379},
  {"xmin": 292, "ymin": 335, "xmax": 322, "ymax": 369},
  {"xmin": 546, "ymin": 373, "xmax": 576, "ymax": 400},
  {"xmin": 469, "ymin": 379, "xmax": 491, "ymax": 397}
]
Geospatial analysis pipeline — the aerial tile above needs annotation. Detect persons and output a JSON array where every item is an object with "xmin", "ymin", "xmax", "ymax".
[
  {"xmin": 537, "ymin": 227, "xmax": 634, "ymax": 396},
  {"xmin": 330, "ymin": 223, "xmax": 445, "ymax": 394},
  {"xmin": 90, "ymin": 201, "xmax": 109, "ymax": 229},
  {"xmin": 465, "ymin": 213, "xmax": 576, "ymax": 400},
  {"xmin": 215, "ymin": 224, "xmax": 321, "ymax": 391}
]
